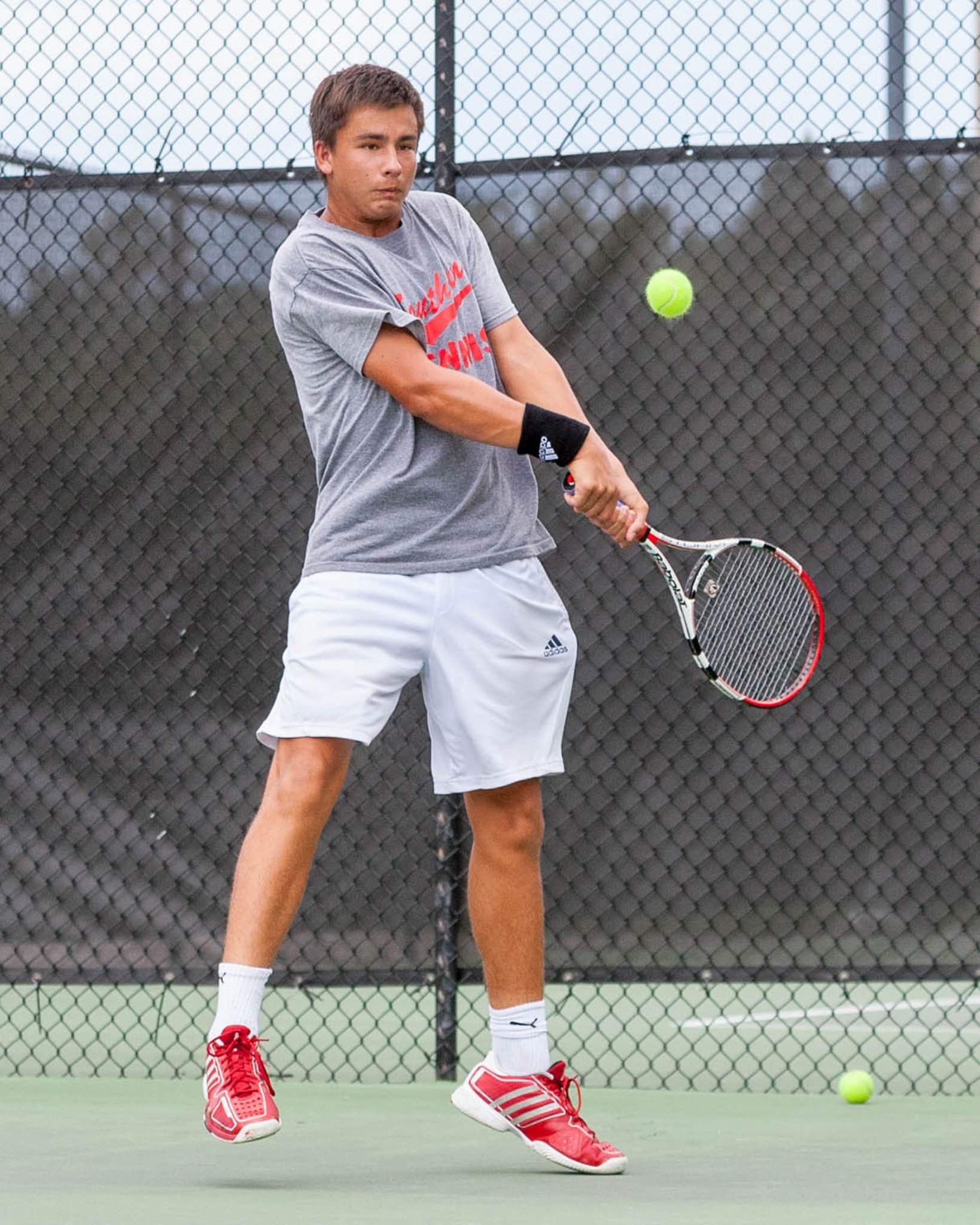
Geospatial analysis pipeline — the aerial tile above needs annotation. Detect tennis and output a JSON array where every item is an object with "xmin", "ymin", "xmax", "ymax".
[
  {"xmin": 645, "ymin": 268, "xmax": 692, "ymax": 318},
  {"xmin": 840, "ymin": 1069, "xmax": 873, "ymax": 1104}
]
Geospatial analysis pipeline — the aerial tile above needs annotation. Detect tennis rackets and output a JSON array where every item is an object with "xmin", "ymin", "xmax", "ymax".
[{"xmin": 559, "ymin": 467, "xmax": 823, "ymax": 708}]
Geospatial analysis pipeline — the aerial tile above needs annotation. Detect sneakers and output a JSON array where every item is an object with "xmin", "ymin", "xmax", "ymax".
[
  {"xmin": 453, "ymin": 1057, "xmax": 627, "ymax": 1175},
  {"xmin": 203, "ymin": 1025, "xmax": 283, "ymax": 1141}
]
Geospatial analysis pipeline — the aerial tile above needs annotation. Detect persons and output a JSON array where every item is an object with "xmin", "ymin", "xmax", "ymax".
[{"xmin": 204, "ymin": 65, "xmax": 650, "ymax": 1175}]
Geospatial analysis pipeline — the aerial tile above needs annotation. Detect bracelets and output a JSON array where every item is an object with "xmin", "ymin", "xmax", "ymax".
[{"xmin": 516, "ymin": 402, "xmax": 590, "ymax": 468}]
